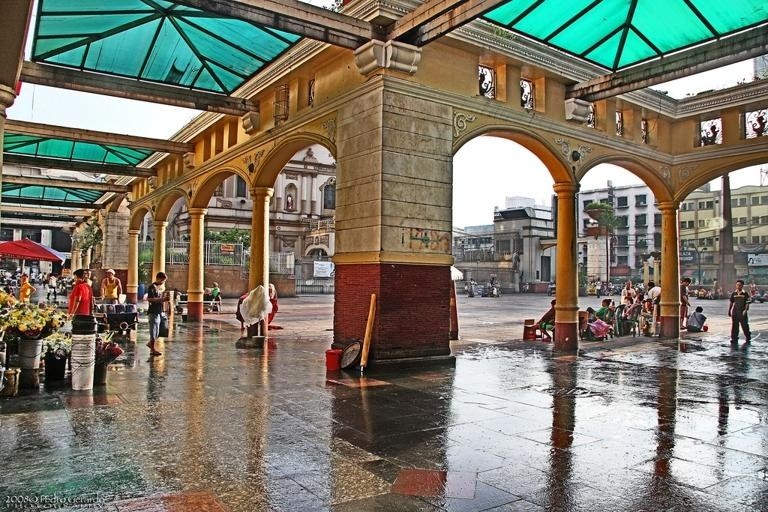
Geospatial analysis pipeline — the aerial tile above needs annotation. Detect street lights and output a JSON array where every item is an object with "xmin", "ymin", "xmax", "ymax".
[
  {"xmin": 743, "ymin": 256, "xmax": 754, "ymax": 279},
  {"xmin": 689, "ymin": 243, "xmax": 707, "ymax": 284},
  {"xmin": 476, "ymin": 256, "xmax": 482, "ymax": 282}
]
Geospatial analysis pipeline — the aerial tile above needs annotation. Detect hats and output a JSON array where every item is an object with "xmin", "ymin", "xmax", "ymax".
[{"xmin": 106, "ymin": 269, "xmax": 115, "ymax": 275}]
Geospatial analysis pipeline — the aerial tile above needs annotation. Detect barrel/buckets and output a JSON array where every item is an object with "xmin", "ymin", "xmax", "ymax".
[
  {"xmin": 72, "ymin": 315, "xmax": 99, "ymax": 391},
  {"xmin": 18, "ymin": 339, "xmax": 43, "ymax": 370},
  {"xmin": 46, "ymin": 356, "xmax": 66, "ymax": 380},
  {"xmin": 94, "ymin": 356, "xmax": 108, "ymax": 385},
  {"xmin": 326, "ymin": 350, "xmax": 342, "ymax": 371}
]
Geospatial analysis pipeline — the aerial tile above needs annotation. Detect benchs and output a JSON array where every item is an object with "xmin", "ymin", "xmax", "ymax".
[{"xmin": 177, "ymin": 294, "xmax": 222, "ymax": 312}]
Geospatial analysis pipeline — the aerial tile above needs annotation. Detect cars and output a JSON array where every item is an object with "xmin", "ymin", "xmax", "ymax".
[{"xmin": 547, "ymin": 280, "xmax": 556, "ymax": 296}]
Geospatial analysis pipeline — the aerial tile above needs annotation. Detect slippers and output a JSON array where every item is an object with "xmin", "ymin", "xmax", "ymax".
[
  {"xmin": 150, "ymin": 351, "xmax": 161, "ymax": 355},
  {"xmin": 147, "ymin": 344, "xmax": 151, "ymax": 348}
]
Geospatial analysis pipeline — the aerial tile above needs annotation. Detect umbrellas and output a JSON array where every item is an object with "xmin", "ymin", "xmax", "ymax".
[{"xmin": 0, "ymin": 237, "xmax": 67, "ymax": 262}]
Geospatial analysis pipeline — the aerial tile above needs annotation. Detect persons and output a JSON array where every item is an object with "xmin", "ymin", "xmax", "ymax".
[
  {"xmin": 208, "ymin": 282, "xmax": 221, "ymax": 312},
  {"xmin": 0, "ymin": 270, "xmax": 179, "ymax": 356},
  {"xmin": 469, "ymin": 276, "xmax": 768, "ymax": 346}
]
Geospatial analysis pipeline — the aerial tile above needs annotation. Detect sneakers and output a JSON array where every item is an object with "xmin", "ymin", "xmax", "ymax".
[
  {"xmin": 746, "ymin": 332, "xmax": 751, "ymax": 340},
  {"xmin": 731, "ymin": 339, "xmax": 738, "ymax": 343}
]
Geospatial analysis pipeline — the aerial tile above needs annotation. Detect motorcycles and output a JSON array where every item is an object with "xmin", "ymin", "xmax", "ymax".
[
  {"xmin": 520, "ymin": 282, "xmax": 531, "ymax": 293},
  {"xmin": 489, "ymin": 284, "xmax": 504, "ymax": 298}
]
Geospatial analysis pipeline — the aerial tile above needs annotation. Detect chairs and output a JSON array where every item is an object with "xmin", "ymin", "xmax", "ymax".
[{"xmin": 523, "ymin": 298, "xmax": 652, "ymax": 344}]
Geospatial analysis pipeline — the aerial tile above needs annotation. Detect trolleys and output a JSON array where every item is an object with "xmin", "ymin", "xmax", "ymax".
[{"xmin": 90, "ymin": 295, "xmax": 139, "ymax": 341}]
